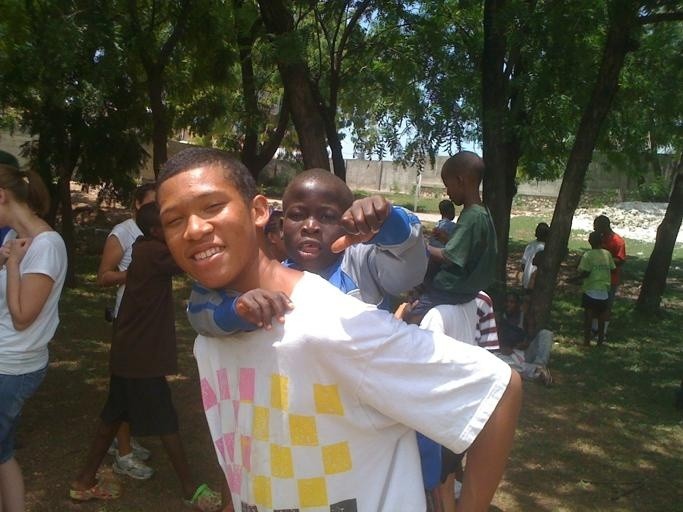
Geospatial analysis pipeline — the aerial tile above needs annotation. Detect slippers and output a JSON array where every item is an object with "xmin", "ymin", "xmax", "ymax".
[
  {"xmin": 182, "ymin": 484, "xmax": 223, "ymax": 511},
  {"xmin": 70, "ymin": 476, "xmax": 124, "ymax": 501}
]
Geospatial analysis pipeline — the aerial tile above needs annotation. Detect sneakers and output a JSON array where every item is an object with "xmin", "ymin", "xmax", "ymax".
[
  {"xmin": 111, "ymin": 449, "xmax": 154, "ymax": 480},
  {"xmin": 108, "ymin": 436, "xmax": 151, "ymax": 461}
]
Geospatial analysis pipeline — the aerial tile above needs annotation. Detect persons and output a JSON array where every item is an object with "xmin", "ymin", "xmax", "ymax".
[
  {"xmin": 517, "ymin": 222, "xmax": 547, "ymax": 332},
  {"xmin": 69, "ymin": 202, "xmax": 228, "ymax": 509},
  {"xmin": 155, "ymin": 144, "xmax": 524, "ymax": 510},
  {"xmin": 186, "ymin": 166, "xmax": 460, "ymax": 512},
  {"xmin": 96, "ymin": 183, "xmax": 158, "ymax": 483},
  {"xmin": 566, "ymin": 215, "xmax": 625, "ymax": 347},
  {"xmin": 0, "ymin": 151, "xmax": 69, "ymax": 512},
  {"xmin": 262, "ymin": 197, "xmax": 290, "ymax": 266}
]
